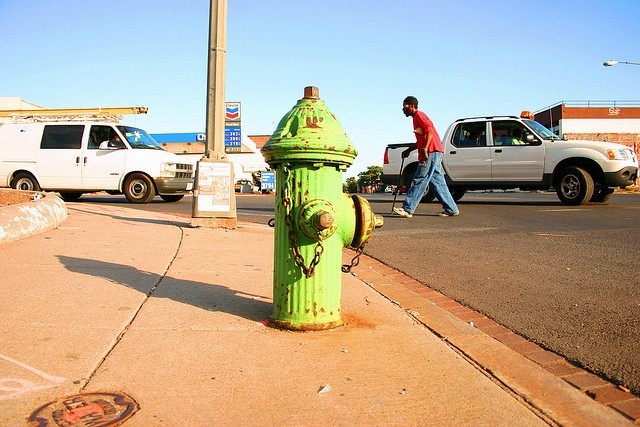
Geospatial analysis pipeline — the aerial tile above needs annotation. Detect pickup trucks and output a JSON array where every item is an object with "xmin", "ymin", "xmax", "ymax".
[{"xmin": 380, "ymin": 114, "xmax": 638, "ymax": 205}]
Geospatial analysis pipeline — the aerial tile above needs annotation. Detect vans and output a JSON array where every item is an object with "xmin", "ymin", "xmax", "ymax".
[{"xmin": 0, "ymin": 118, "xmax": 194, "ymax": 204}]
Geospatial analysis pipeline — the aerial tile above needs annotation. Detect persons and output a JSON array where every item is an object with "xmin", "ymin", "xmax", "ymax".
[
  {"xmin": 510, "ymin": 131, "xmax": 525, "ymax": 144},
  {"xmin": 97, "ymin": 135, "xmax": 120, "ymax": 148},
  {"xmin": 393, "ymin": 96, "xmax": 459, "ymax": 217}
]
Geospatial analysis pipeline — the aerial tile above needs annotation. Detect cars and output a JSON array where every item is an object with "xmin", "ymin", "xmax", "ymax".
[{"xmin": 385, "ymin": 186, "xmax": 393, "ymax": 193}]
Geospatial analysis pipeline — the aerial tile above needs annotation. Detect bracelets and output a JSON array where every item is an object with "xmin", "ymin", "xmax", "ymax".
[{"xmin": 422, "ymin": 147, "xmax": 428, "ymax": 151}]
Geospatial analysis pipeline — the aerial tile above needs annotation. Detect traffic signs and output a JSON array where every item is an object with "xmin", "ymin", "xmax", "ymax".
[{"xmin": 225, "ymin": 126, "xmax": 241, "ymax": 147}]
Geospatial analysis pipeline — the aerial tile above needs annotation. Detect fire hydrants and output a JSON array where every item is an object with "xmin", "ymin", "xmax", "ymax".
[{"xmin": 260, "ymin": 86, "xmax": 384, "ymax": 332}]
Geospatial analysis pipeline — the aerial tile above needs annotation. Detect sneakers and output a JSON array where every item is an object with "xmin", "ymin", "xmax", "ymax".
[
  {"xmin": 437, "ymin": 209, "xmax": 459, "ymax": 216},
  {"xmin": 394, "ymin": 207, "xmax": 412, "ymax": 217}
]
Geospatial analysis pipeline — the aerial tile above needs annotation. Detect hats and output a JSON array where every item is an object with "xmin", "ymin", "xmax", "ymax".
[{"xmin": 404, "ymin": 96, "xmax": 418, "ymax": 106}]
